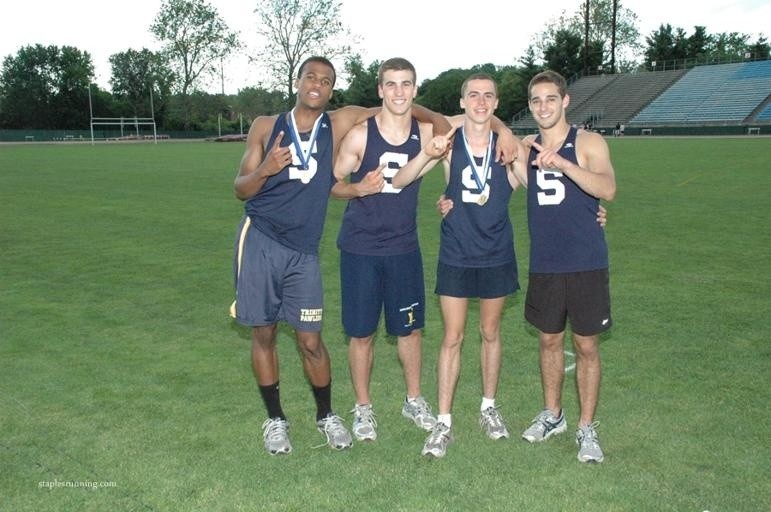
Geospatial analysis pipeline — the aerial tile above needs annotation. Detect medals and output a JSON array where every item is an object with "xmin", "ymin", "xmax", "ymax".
[
  {"xmin": 298, "ymin": 171, "xmax": 311, "ymax": 185},
  {"xmin": 478, "ymin": 194, "xmax": 487, "ymax": 204}
]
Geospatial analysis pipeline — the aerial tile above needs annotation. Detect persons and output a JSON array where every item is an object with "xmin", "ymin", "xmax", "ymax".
[
  {"xmin": 228, "ymin": 56, "xmax": 453, "ymax": 458},
  {"xmin": 433, "ymin": 69, "xmax": 618, "ymax": 465},
  {"xmin": 389, "ymin": 71, "xmax": 609, "ymax": 460},
  {"xmin": 330, "ymin": 57, "xmax": 520, "ymax": 445}
]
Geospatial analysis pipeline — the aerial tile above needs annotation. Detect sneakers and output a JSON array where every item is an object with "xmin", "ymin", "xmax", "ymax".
[
  {"xmin": 402, "ymin": 395, "xmax": 438, "ymax": 432},
  {"xmin": 479, "ymin": 406, "xmax": 509, "ymax": 441},
  {"xmin": 421, "ymin": 421, "xmax": 453, "ymax": 457},
  {"xmin": 263, "ymin": 418, "xmax": 293, "ymax": 457},
  {"xmin": 575, "ymin": 423, "xmax": 605, "ymax": 465},
  {"xmin": 316, "ymin": 414, "xmax": 353, "ymax": 452},
  {"xmin": 353, "ymin": 403, "xmax": 378, "ymax": 442},
  {"xmin": 521, "ymin": 407, "xmax": 568, "ymax": 445}
]
